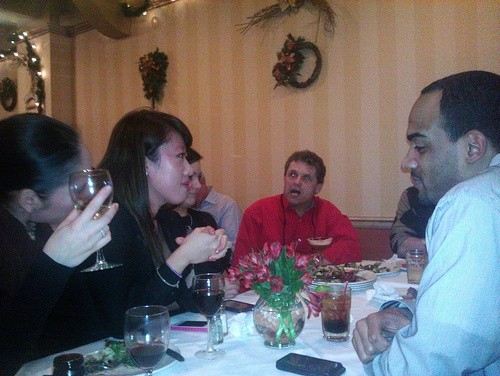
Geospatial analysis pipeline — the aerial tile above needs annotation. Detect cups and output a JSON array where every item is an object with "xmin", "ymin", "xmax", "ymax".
[
  {"xmin": 406, "ymin": 248, "xmax": 426, "ymax": 284},
  {"xmin": 321, "ymin": 285, "xmax": 352, "ymax": 342}
]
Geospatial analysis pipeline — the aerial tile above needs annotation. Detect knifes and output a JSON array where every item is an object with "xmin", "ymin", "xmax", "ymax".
[{"xmin": 166, "ymin": 348, "xmax": 185, "ymax": 362}]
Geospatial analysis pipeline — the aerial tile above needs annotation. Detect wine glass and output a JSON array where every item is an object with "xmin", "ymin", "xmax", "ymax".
[
  {"xmin": 192, "ymin": 273, "xmax": 225, "ymax": 360},
  {"xmin": 69, "ymin": 170, "xmax": 123, "ymax": 272},
  {"xmin": 307, "ymin": 237, "xmax": 334, "ymax": 267}
]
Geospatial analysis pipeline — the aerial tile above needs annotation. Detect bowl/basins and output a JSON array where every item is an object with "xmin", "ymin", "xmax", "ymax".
[{"xmin": 312, "ymin": 267, "xmax": 377, "ymax": 292}]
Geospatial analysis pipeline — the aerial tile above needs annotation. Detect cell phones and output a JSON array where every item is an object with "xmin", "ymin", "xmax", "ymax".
[
  {"xmin": 223, "ymin": 300, "xmax": 254, "ymax": 313},
  {"xmin": 275, "ymin": 353, "xmax": 345, "ymax": 376},
  {"xmin": 171, "ymin": 320, "xmax": 208, "ymax": 332}
]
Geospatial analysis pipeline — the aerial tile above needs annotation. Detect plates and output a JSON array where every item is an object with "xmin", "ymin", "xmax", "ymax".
[
  {"xmin": 339, "ymin": 262, "xmax": 408, "ymax": 275},
  {"xmin": 35, "ymin": 345, "xmax": 181, "ymax": 376}
]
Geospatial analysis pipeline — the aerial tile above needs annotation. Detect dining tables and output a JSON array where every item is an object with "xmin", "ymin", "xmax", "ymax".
[{"xmin": 15, "ymin": 260, "xmax": 424, "ymax": 376}]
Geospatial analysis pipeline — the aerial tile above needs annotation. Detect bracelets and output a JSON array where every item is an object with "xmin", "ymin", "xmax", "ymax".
[{"xmin": 155, "ymin": 265, "xmax": 180, "ymax": 289}]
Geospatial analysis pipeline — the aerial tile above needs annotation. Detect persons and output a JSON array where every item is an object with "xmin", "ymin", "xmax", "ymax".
[
  {"xmin": 154, "ymin": 144, "xmax": 231, "ymax": 311},
  {"xmin": 351, "ymin": 72, "xmax": 500, "ymax": 376},
  {"xmin": 229, "ymin": 150, "xmax": 361, "ymax": 295},
  {"xmin": 0, "ymin": 112, "xmax": 140, "ymax": 376},
  {"xmin": 188, "ymin": 171, "xmax": 242, "ymax": 243},
  {"xmin": 92, "ymin": 106, "xmax": 233, "ymax": 338},
  {"xmin": 388, "ymin": 187, "xmax": 436, "ymax": 259}
]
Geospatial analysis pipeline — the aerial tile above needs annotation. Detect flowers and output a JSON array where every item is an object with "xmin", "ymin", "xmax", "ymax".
[
  {"xmin": 271, "ymin": 33, "xmax": 305, "ymax": 88},
  {"xmin": 139, "ymin": 47, "xmax": 170, "ymax": 109},
  {"xmin": 226, "ymin": 241, "xmax": 331, "ymax": 343},
  {"xmin": 0, "ymin": 76, "xmax": 16, "ymax": 99}
]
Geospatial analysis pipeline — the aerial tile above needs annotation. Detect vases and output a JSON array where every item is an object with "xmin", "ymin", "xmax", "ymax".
[{"xmin": 253, "ymin": 292, "xmax": 306, "ymax": 349}]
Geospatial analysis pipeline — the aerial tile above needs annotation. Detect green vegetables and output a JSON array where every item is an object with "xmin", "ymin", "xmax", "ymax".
[
  {"xmin": 345, "ymin": 262, "xmax": 390, "ymax": 274},
  {"xmin": 83, "ymin": 340, "xmax": 139, "ymax": 374}
]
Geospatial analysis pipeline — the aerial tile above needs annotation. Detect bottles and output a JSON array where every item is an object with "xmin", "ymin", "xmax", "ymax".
[
  {"xmin": 210, "ymin": 305, "xmax": 228, "ymax": 345},
  {"xmin": 54, "ymin": 353, "xmax": 85, "ymax": 376},
  {"xmin": 124, "ymin": 305, "xmax": 171, "ymax": 376}
]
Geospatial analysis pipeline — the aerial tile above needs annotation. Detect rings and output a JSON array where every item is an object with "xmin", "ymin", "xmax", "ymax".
[{"xmin": 99, "ymin": 229, "xmax": 106, "ymax": 238}]
